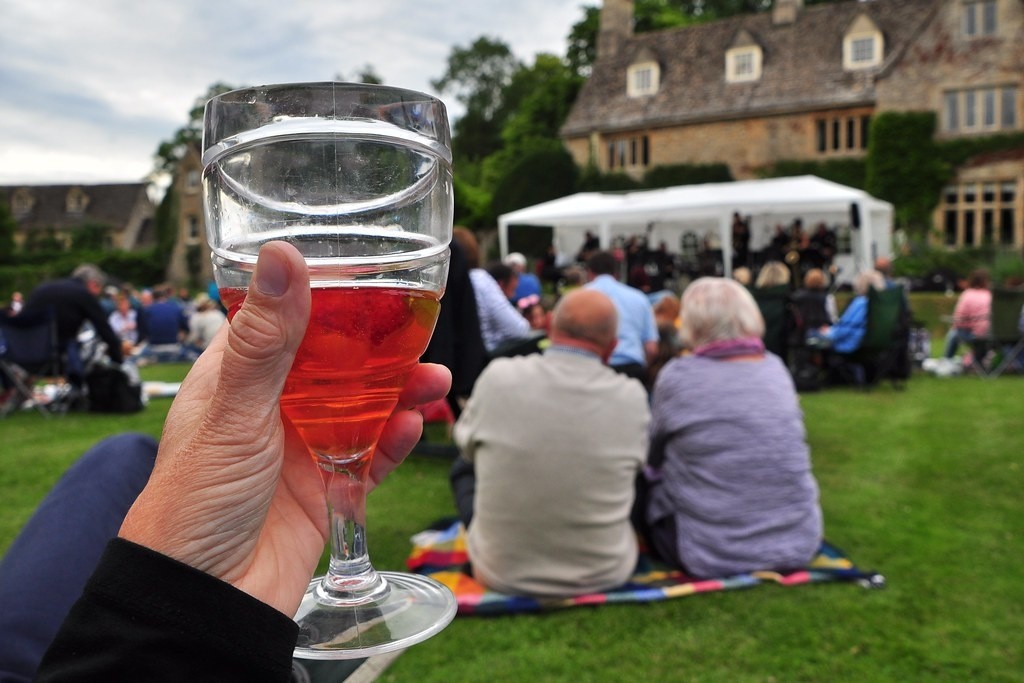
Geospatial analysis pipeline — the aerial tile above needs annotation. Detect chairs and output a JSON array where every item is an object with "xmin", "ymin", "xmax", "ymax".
[
  {"xmin": 785, "ymin": 289, "xmax": 834, "ymax": 366},
  {"xmin": 0, "ymin": 304, "xmax": 101, "ymax": 420},
  {"xmin": 950, "ymin": 286, "xmax": 1024, "ymax": 383},
  {"xmin": 807, "ymin": 282, "xmax": 927, "ymax": 394}
]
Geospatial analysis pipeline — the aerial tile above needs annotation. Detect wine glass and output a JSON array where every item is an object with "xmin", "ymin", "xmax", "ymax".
[{"xmin": 200, "ymin": 82, "xmax": 459, "ymax": 660}]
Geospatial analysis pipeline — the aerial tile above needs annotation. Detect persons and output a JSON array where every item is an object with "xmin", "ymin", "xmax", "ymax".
[
  {"xmin": 1, "ymin": 210, "xmax": 1024, "ymax": 415},
  {"xmin": 0, "ymin": 239, "xmax": 453, "ymax": 681},
  {"xmin": 451, "ymin": 277, "xmax": 825, "ymax": 596}
]
